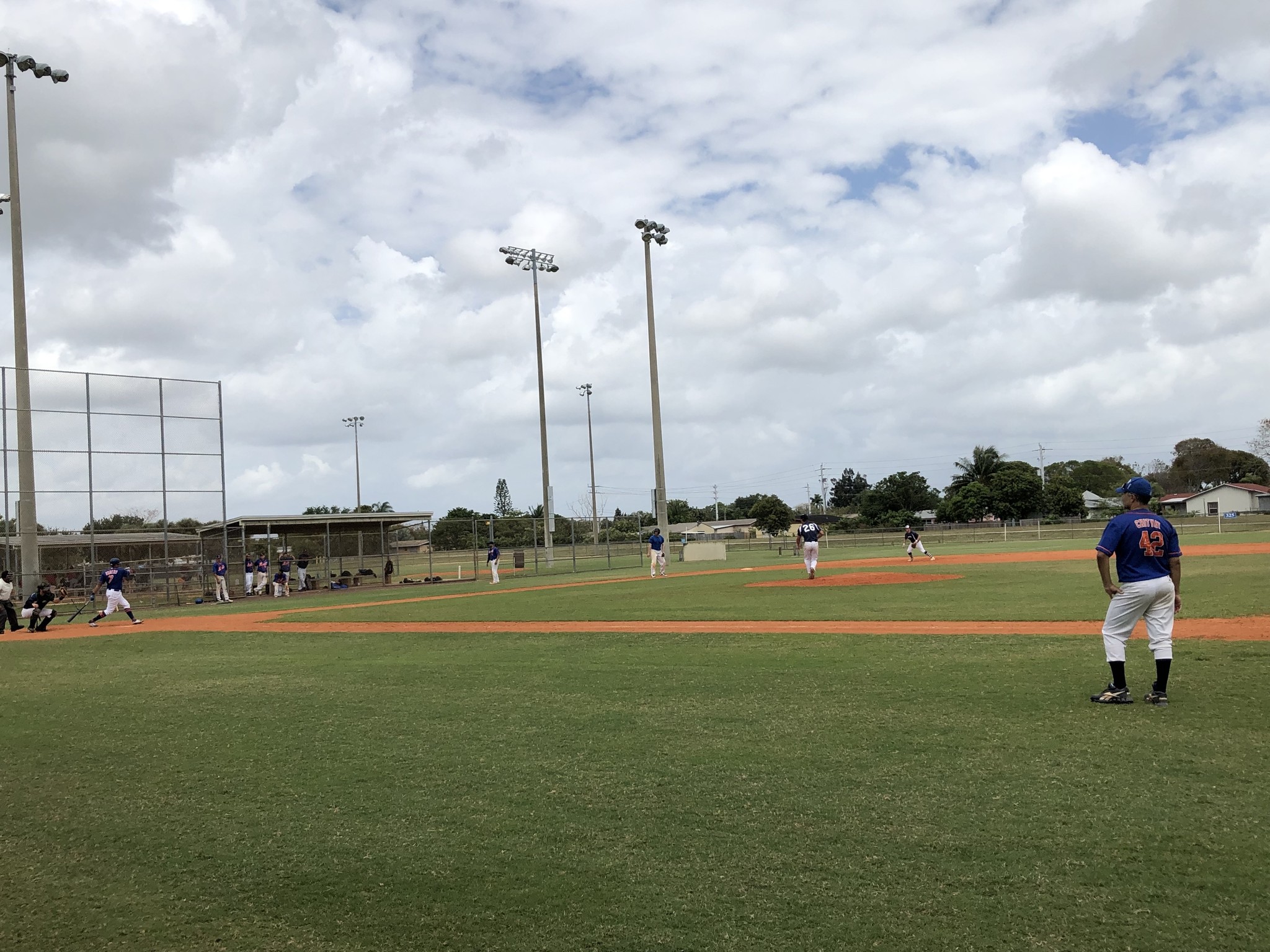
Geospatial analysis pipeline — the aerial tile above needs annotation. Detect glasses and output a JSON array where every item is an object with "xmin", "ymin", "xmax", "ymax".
[
  {"xmin": 247, "ymin": 556, "xmax": 250, "ymax": 557},
  {"xmin": 1121, "ymin": 483, "xmax": 1136, "ymax": 495}
]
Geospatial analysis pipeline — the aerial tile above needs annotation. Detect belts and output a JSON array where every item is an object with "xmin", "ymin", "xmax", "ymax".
[{"xmin": 108, "ymin": 589, "xmax": 119, "ymax": 591}]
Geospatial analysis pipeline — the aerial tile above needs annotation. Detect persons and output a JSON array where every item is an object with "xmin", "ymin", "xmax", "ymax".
[
  {"xmin": 297, "ymin": 549, "xmax": 311, "ymax": 592},
  {"xmin": 212, "ymin": 555, "xmax": 234, "ymax": 603},
  {"xmin": 647, "ymin": 528, "xmax": 668, "ymax": 577},
  {"xmin": 1089, "ymin": 477, "xmax": 1182, "ymax": 704},
  {"xmin": 902, "ymin": 525, "xmax": 935, "ymax": 561},
  {"xmin": 0, "ymin": 559, "xmax": 207, "ymax": 597},
  {"xmin": 277, "ymin": 553, "xmax": 295, "ymax": 593},
  {"xmin": 796, "ymin": 514, "xmax": 824, "ymax": 579},
  {"xmin": 0, "ymin": 571, "xmax": 25, "ymax": 634},
  {"xmin": 88, "ymin": 558, "xmax": 144, "ymax": 628},
  {"xmin": 272, "ymin": 569, "xmax": 290, "ymax": 598},
  {"xmin": 245, "ymin": 552, "xmax": 269, "ymax": 596},
  {"xmin": 21, "ymin": 582, "xmax": 68, "ymax": 633},
  {"xmin": 487, "ymin": 541, "xmax": 501, "ymax": 584}
]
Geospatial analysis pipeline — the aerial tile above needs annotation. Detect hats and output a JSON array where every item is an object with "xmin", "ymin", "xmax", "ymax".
[
  {"xmin": 217, "ymin": 555, "xmax": 221, "ymax": 558},
  {"xmin": 1115, "ymin": 476, "xmax": 1152, "ymax": 496},
  {"xmin": 486, "ymin": 542, "xmax": 493, "ymax": 545},
  {"xmin": 905, "ymin": 525, "xmax": 910, "ymax": 528}
]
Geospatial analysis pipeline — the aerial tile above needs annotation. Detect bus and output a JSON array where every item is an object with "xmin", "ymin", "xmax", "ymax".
[{"xmin": 181, "ymin": 555, "xmax": 209, "ymax": 565}]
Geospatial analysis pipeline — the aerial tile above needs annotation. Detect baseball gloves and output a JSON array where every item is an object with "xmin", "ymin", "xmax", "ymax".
[
  {"xmin": 59, "ymin": 587, "xmax": 68, "ymax": 598},
  {"xmin": 661, "ymin": 552, "xmax": 665, "ymax": 557},
  {"xmin": 911, "ymin": 542, "xmax": 917, "ymax": 549}
]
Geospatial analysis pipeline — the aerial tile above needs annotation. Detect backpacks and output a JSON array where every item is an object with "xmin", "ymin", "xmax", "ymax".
[{"xmin": 384, "ymin": 561, "xmax": 394, "ymax": 579}]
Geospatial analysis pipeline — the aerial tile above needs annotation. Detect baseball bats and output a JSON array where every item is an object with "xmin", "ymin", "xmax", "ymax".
[{"xmin": 68, "ymin": 600, "xmax": 91, "ymax": 622}]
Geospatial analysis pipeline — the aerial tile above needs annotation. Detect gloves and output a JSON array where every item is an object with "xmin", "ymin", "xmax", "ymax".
[{"xmin": 90, "ymin": 592, "xmax": 95, "ymax": 601}]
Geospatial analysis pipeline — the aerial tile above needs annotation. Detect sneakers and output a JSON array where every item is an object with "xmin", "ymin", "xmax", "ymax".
[
  {"xmin": 88, "ymin": 620, "xmax": 100, "ymax": 627},
  {"xmin": 1090, "ymin": 682, "xmax": 1169, "ymax": 706},
  {"xmin": 131, "ymin": 619, "xmax": 144, "ymax": 625},
  {"xmin": 651, "ymin": 573, "xmax": 667, "ymax": 577}
]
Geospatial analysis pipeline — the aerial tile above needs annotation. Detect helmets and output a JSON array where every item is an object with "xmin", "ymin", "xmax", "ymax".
[
  {"xmin": 110, "ymin": 558, "xmax": 120, "ymax": 564},
  {"xmin": 654, "ymin": 528, "xmax": 660, "ymax": 534},
  {"xmin": 195, "ymin": 598, "xmax": 203, "ymax": 604}
]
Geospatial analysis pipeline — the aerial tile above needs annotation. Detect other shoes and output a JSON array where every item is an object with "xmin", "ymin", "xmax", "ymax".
[
  {"xmin": 286, "ymin": 595, "xmax": 290, "ymax": 596},
  {"xmin": 930, "ymin": 557, "xmax": 935, "ymax": 560},
  {"xmin": 275, "ymin": 595, "xmax": 277, "ymax": 597},
  {"xmin": 489, "ymin": 581, "xmax": 499, "ymax": 584},
  {"xmin": 218, "ymin": 599, "xmax": 234, "ymax": 602},
  {"xmin": 907, "ymin": 557, "xmax": 914, "ymax": 561},
  {"xmin": 28, "ymin": 626, "xmax": 46, "ymax": 633},
  {"xmin": 246, "ymin": 589, "xmax": 262, "ymax": 596},
  {"xmin": 809, "ymin": 569, "xmax": 815, "ymax": 579},
  {"xmin": 298, "ymin": 587, "xmax": 306, "ymax": 592},
  {"xmin": 0, "ymin": 625, "xmax": 24, "ymax": 634}
]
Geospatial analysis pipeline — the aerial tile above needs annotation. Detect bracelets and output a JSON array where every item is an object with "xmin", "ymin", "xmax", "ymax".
[
  {"xmin": 903, "ymin": 543, "xmax": 905, "ymax": 546},
  {"xmin": 59, "ymin": 596, "xmax": 64, "ymax": 600},
  {"xmin": 915, "ymin": 540, "xmax": 918, "ymax": 542}
]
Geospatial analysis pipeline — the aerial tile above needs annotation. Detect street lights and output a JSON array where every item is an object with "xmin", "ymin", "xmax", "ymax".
[
  {"xmin": 501, "ymin": 247, "xmax": 561, "ymax": 564},
  {"xmin": 635, "ymin": 219, "xmax": 670, "ymax": 559},
  {"xmin": 0, "ymin": 51, "xmax": 68, "ymax": 599},
  {"xmin": 343, "ymin": 416, "xmax": 366, "ymax": 582},
  {"xmin": 578, "ymin": 383, "xmax": 599, "ymax": 545}
]
